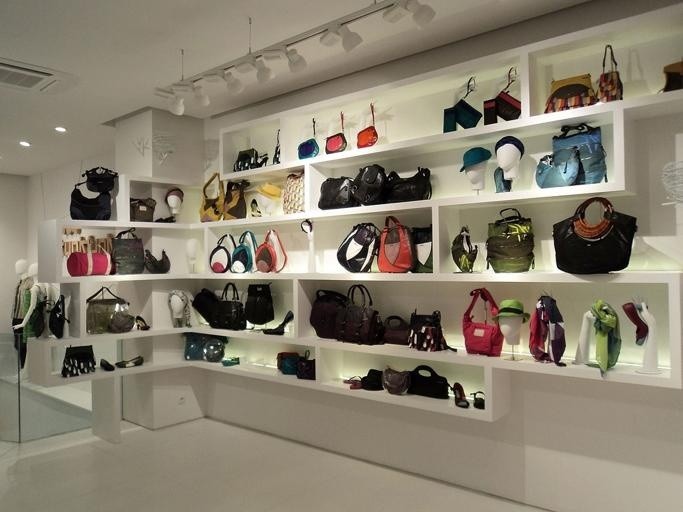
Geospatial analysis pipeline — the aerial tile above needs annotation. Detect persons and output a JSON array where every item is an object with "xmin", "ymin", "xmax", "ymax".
[
  {"xmin": 491, "ymin": 298, "xmax": 530, "ymax": 346},
  {"xmin": 167, "ymin": 288, "xmax": 192, "ymax": 328},
  {"xmin": 494, "ymin": 136, "xmax": 525, "ymax": 181},
  {"xmin": 164, "ymin": 187, "xmax": 185, "ymax": 215},
  {"xmin": 458, "ymin": 146, "xmax": 493, "ymax": 192},
  {"xmin": 185, "ymin": 238, "xmax": 200, "ymax": 266},
  {"xmin": 11, "ymin": 263, "xmax": 38, "ymax": 369},
  {"xmin": 11, "ymin": 258, "xmax": 34, "ymax": 349}
]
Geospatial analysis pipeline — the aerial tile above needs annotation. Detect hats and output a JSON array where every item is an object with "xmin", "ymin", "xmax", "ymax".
[
  {"xmin": 492, "ymin": 299, "xmax": 530, "ymax": 323},
  {"xmin": 460, "ymin": 147, "xmax": 492, "ymax": 172},
  {"xmin": 257, "ymin": 182, "xmax": 281, "ymax": 201},
  {"xmin": 165, "ymin": 187, "xmax": 184, "ymax": 205},
  {"xmin": 495, "ymin": 136, "xmax": 524, "ymax": 159}
]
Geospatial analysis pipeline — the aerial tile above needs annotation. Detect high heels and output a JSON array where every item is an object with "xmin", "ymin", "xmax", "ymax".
[
  {"xmin": 251, "ymin": 199, "xmax": 262, "ymax": 217},
  {"xmin": 257, "ymin": 154, "xmax": 269, "ymax": 168},
  {"xmin": 623, "ymin": 303, "xmax": 648, "ymax": 345},
  {"xmin": 136, "ymin": 316, "xmax": 150, "ymax": 330},
  {"xmin": 263, "ymin": 311, "xmax": 294, "ymax": 334},
  {"xmin": 451, "ymin": 383, "xmax": 469, "ymax": 408}
]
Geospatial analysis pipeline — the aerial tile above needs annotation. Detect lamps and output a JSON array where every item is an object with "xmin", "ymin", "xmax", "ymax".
[
  {"xmin": 168, "ymin": 95, "xmax": 185, "ymax": 118},
  {"xmin": 335, "ymin": 25, "xmax": 364, "ymax": 53},
  {"xmin": 404, "ymin": 0, "xmax": 436, "ymax": 27},
  {"xmin": 222, "ymin": 72, "xmax": 242, "ymax": 95},
  {"xmin": 285, "ymin": 49, "xmax": 308, "ymax": 74},
  {"xmin": 255, "ymin": 54, "xmax": 276, "ymax": 85},
  {"xmin": 192, "ymin": 86, "xmax": 210, "ymax": 108}
]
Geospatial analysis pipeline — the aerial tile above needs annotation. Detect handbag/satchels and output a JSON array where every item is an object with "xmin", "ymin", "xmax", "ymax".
[
  {"xmin": 210, "ymin": 230, "xmax": 287, "ymax": 273},
  {"xmin": 277, "ymin": 350, "xmax": 315, "ymax": 380},
  {"xmin": 298, "ymin": 103, "xmax": 378, "ymax": 159},
  {"xmin": 130, "ymin": 198, "xmax": 156, "ymax": 221},
  {"xmin": 283, "ymin": 170, "xmax": 304, "ymax": 214},
  {"xmin": 192, "ymin": 282, "xmax": 274, "ymax": 330},
  {"xmin": 62, "ymin": 345, "xmax": 96, "ymax": 377},
  {"xmin": 70, "ymin": 167, "xmax": 119, "ymax": 220},
  {"xmin": 310, "ymin": 285, "xmax": 458, "ymax": 352},
  {"xmin": 181, "ymin": 333, "xmax": 228, "ymax": 362},
  {"xmin": 657, "ymin": 61, "xmax": 683, "ymax": 93},
  {"xmin": 463, "ymin": 288, "xmax": 503, "ymax": 356},
  {"xmin": 599, "ymin": 45, "xmax": 623, "ymax": 101},
  {"xmin": 535, "ymin": 123, "xmax": 608, "ymax": 188},
  {"xmin": 273, "ymin": 130, "xmax": 280, "ymax": 165},
  {"xmin": 545, "ymin": 73, "xmax": 599, "ymax": 113},
  {"xmin": 233, "ymin": 148, "xmax": 258, "ymax": 172},
  {"xmin": 318, "ymin": 165, "xmax": 432, "ymax": 210},
  {"xmin": 67, "ymin": 228, "xmax": 170, "ymax": 276},
  {"xmin": 487, "ymin": 207, "xmax": 534, "ymax": 273},
  {"xmin": 199, "ymin": 173, "xmax": 250, "ymax": 222},
  {"xmin": 337, "ymin": 216, "xmax": 432, "ymax": 273},
  {"xmin": 452, "ymin": 227, "xmax": 477, "ymax": 273},
  {"xmin": 444, "ymin": 68, "xmax": 521, "ymax": 132},
  {"xmin": 87, "ymin": 287, "xmax": 135, "ymax": 333},
  {"xmin": 361, "ymin": 365, "xmax": 450, "ymax": 399},
  {"xmin": 553, "ymin": 197, "xmax": 637, "ymax": 274}
]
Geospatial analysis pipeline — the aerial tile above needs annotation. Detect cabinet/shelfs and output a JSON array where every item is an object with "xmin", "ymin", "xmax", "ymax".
[
  {"xmin": 27, "ymin": 176, "xmax": 204, "ymax": 432},
  {"xmin": 204, "ymin": 4, "xmax": 682, "ymax": 424}
]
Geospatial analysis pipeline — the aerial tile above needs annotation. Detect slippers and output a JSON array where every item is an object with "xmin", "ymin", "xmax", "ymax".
[{"xmin": 344, "ymin": 376, "xmax": 361, "ymax": 383}]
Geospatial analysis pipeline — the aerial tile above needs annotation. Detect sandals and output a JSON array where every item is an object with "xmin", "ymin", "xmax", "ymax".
[
  {"xmin": 471, "ymin": 391, "xmax": 485, "ymax": 409},
  {"xmin": 155, "ymin": 216, "xmax": 176, "ymax": 222}
]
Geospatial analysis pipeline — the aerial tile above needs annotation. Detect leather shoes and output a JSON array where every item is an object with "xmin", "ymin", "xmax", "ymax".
[
  {"xmin": 101, "ymin": 359, "xmax": 114, "ymax": 371},
  {"xmin": 222, "ymin": 357, "xmax": 239, "ymax": 366},
  {"xmin": 116, "ymin": 356, "xmax": 143, "ymax": 368}
]
